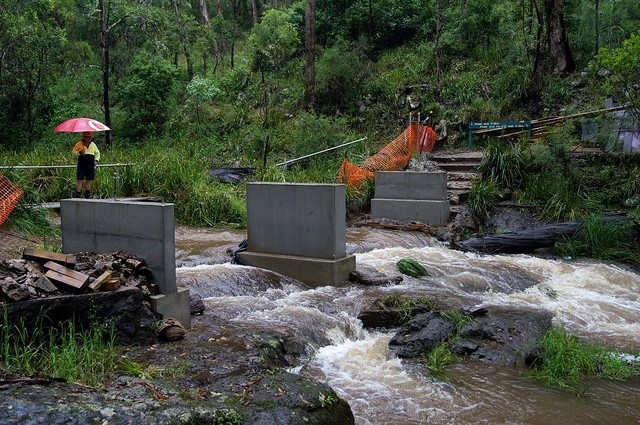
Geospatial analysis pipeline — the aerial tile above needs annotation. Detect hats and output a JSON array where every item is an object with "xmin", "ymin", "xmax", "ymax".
[{"xmin": 82, "ymin": 132, "xmax": 92, "ymax": 136}]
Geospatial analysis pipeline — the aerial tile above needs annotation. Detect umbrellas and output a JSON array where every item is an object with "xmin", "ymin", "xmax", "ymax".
[{"xmin": 53, "ymin": 113, "xmax": 112, "ymax": 149}]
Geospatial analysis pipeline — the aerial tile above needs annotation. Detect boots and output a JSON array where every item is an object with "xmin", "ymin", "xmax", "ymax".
[
  {"xmin": 85, "ymin": 190, "xmax": 90, "ymax": 199},
  {"xmin": 76, "ymin": 189, "xmax": 82, "ymax": 198}
]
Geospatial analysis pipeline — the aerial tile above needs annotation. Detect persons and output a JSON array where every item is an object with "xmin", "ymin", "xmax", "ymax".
[{"xmin": 72, "ymin": 131, "xmax": 101, "ymax": 199}]
